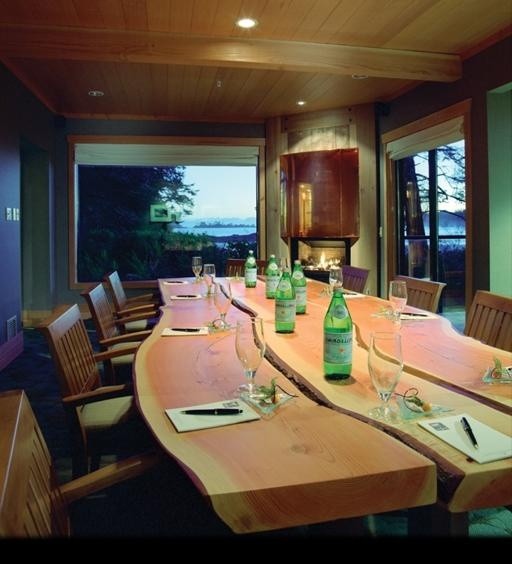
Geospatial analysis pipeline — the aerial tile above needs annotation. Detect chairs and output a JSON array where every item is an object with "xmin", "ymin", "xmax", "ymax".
[
  {"xmin": 225, "ymin": 259, "xmax": 266, "ymax": 277},
  {"xmin": 464, "ymin": 290, "xmax": 512, "ymax": 352},
  {"xmin": 396, "ymin": 275, "xmax": 447, "ymax": 313},
  {"xmin": 37, "ymin": 302, "xmax": 140, "ymax": 498},
  {"xmin": 342, "ymin": 266, "xmax": 369, "ymax": 294},
  {"xmin": 1, "ymin": 389, "xmax": 160, "ymax": 538},
  {"xmin": 102, "ymin": 270, "xmax": 155, "ymax": 335},
  {"xmin": 80, "ymin": 282, "xmax": 158, "ymax": 385}
]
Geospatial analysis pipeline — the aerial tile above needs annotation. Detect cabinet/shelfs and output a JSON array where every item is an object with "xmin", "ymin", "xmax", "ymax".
[{"xmin": 280, "ymin": 147, "xmax": 360, "ymax": 246}]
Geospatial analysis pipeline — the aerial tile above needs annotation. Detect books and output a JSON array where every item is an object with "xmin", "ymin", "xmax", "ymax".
[
  {"xmin": 416, "ymin": 413, "xmax": 512, "ymax": 464},
  {"xmin": 162, "ymin": 280, "xmax": 188, "ymax": 285},
  {"xmin": 343, "ymin": 292, "xmax": 365, "ymax": 299},
  {"xmin": 400, "ymin": 312, "xmax": 439, "ymax": 320},
  {"xmin": 170, "ymin": 294, "xmax": 202, "ymax": 299},
  {"xmin": 161, "ymin": 327, "xmax": 208, "ymax": 336},
  {"xmin": 165, "ymin": 397, "xmax": 261, "ymax": 433}
]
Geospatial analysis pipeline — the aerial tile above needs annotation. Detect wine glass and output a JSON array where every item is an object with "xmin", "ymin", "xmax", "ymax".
[
  {"xmin": 213, "ymin": 282, "xmax": 234, "ymax": 329},
  {"xmin": 389, "ymin": 281, "xmax": 408, "ymax": 329},
  {"xmin": 235, "ymin": 317, "xmax": 268, "ymax": 403},
  {"xmin": 204, "ymin": 265, "xmax": 216, "ymax": 295},
  {"xmin": 329, "ymin": 268, "xmax": 343, "ymax": 291},
  {"xmin": 277, "ymin": 258, "xmax": 287, "ymax": 273},
  {"xmin": 192, "ymin": 258, "xmax": 203, "ymax": 285},
  {"xmin": 368, "ymin": 333, "xmax": 402, "ymax": 426}
]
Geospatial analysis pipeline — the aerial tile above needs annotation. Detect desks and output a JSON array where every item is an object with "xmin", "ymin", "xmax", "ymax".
[{"xmin": 133, "ymin": 274, "xmax": 512, "ymax": 538}]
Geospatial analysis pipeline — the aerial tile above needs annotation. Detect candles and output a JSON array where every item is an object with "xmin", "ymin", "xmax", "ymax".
[{"xmin": 319, "ymin": 252, "xmax": 326, "ymax": 267}]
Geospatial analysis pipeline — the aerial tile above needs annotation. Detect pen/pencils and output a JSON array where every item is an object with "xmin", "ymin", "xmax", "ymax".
[
  {"xmin": 460, "ymin": 416, "xmax": 479, "ymax": 451},
  {"xmin": 180, "ymin": 408, "xmax": 242, "ymax": 414},
  {"xmin": 166, "ymin": 280, "xmax": 184, "ymax": 283},
  {"xmin": 176, "ymin": 294, "xmax": 197, "ymax": 297},
  {"xmin": 342, "ymin": 291, "xmax": 358, "ymax": 296},
  {"xmin": 399, "ymin": 311, "xmax": 428, "ymax": 317},
  {"xmin": 171, "ymin": 327, "xmax": 199, "ymax": 331}
]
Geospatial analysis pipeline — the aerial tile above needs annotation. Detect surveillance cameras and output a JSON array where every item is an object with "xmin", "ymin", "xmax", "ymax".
[{"xmin": 216, "ymin": 80, "xmax": 224, "ymax": 88}]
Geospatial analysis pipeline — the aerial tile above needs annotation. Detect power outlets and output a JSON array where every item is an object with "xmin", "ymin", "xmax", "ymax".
[
  {"xmin": 13, "ymin": 208, "xmax": 20, "ymax": 220},
  {"xmin": 7, "ymin": 207, "xmax": 12, "ymax": 220}
]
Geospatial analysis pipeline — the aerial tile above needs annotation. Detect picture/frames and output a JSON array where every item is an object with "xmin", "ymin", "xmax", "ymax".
[{"xmin": 150, "ymin": 204, "xmax": 172, "ymax": 222}]
